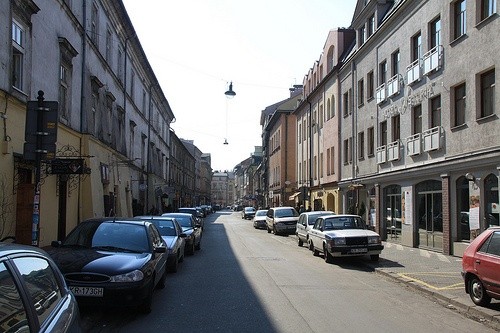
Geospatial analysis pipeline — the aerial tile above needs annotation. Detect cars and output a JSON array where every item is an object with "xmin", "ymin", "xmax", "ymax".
[
  {"xmin": 253, "ymin": 210, "xmax": 269, "ymax": 229},
  {"xmin": 266, "ymin": 207, "xmax": 300, "ymax": 235},
  {"xmin": 295, "ymin": 210, "xmax": 347, "ymax": 251},
  {"xmin": 47, "ymin": 217, "xmax": 167, "ymax": 314},
  {"xmin": 241, "ymin": 207, "xmax": 256, "ymax": 220},
  {"xmin": 307, "ymin": 214, "xmax": 385, "ymax": 264},
  {"xmin": 160, "ymin": 213, "xmax": 202, "ymax": 256},
  {"xmin": 134, "ymin": 216, "xmax": 187, "ymax": 273},
  {"xmin": 460, "ymin": 226, "xmax": 500, "ymax": 308},
  {"xmin": 195, "ymin": 205, "xmax": 213, "ymax": 218},
  {"xmin": 0, "ymin": 243, "xmax": 82, "ymax": 333},
  {"xmin": 177, "ymin": 208, "xmax": 205, "ymax": 231}
]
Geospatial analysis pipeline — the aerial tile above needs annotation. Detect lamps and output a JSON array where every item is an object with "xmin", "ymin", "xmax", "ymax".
[
  {"xmin": 224, "ymin": 139, "xmax": 228, "ymax": 144},
  {"xmin": 130, "ymin": 179, "xmax": 147, "ymax": 192},
  {"xmin": 224, "ymin": 82, "xmax": 236, "ymax": 96},
  {"xmin": 465, "ymin": 173, "xmax": 476, "ymax": 182}
]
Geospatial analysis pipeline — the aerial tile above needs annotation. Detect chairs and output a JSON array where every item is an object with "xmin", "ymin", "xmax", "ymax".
[
  {"xmin": 342, "ymin": 222, "xmax": 352, "ymax": 228},
  {"xmin": 323, "ymin": 221, "xmax": 333, "ymax": 230}
]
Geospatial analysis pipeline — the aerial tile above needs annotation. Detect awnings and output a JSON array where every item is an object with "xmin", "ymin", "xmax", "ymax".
[{"xmin": 289, "ymin": 192, "xmax": 301, "ymax": 200}]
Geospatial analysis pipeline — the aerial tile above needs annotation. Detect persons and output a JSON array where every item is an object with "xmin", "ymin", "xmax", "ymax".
[
  {"xmin": 253, "ymin": 202, "xmax": 258, "ymax": 210},
  {"xmin": 295, "ymin": 204, "xmax": 332, "ymax": 215},
  {"xmin": 266, "ymin": 204, "xmax": 274, "ymax": 210},
  {"xmin": 150, "ymin": 203, "xmax": 172, "ymax": 216}
]
